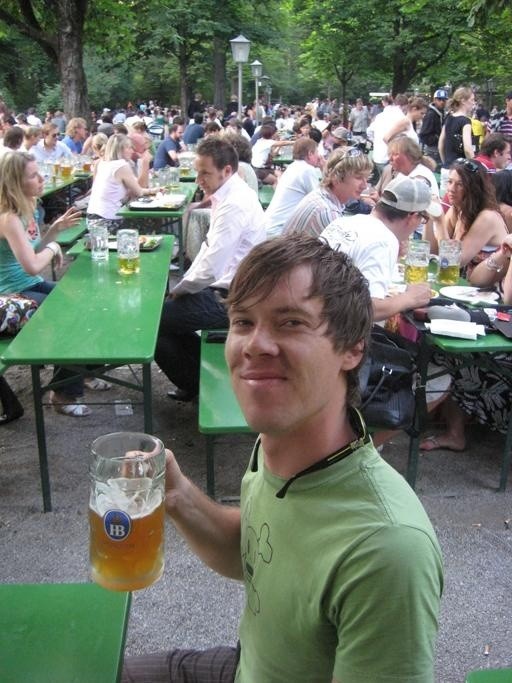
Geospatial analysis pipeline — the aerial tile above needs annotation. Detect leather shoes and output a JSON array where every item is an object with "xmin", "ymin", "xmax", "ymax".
[{"xmin": 167, "ymin": 385, "xmax": 194, "ymax": 401}]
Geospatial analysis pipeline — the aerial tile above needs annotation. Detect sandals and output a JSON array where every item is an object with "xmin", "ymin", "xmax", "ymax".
[
  {"xmin": 420, "ymin": 434, "xmax": 465, "ymax": 453},
  {"xmin": 84, "ymin": 378, "xmax": 113, "ymax": 392},
  {"xmin": 49, "ymin": 398, "xmax": 90, "ymax": 417}
]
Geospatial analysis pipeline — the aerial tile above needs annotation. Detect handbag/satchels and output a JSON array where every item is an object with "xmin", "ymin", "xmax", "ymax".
[
  {"xmin": 452, "ymin": 130, "xmax": 468, "ymax": 154},
  {"xmin": 0, "ymin": 292, "xmax": 38, "ymax": 338},
  {"xmin": 351, "ymin": 324, "xmax": 419, "ymax": 428}
]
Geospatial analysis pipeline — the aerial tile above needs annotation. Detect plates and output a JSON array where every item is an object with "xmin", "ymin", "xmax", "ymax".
[
  {"xmin": 441, "ymin": 286, "xmax": 501, "ymax": 303},
  {"xmin": 389, "ymin": 286, "xmax": 439, "ymax": 300}
]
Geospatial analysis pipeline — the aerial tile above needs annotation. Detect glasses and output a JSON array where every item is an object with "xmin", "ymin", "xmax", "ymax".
[
  {"xmin": 455, "ymin": 157, "xmax": 481, "ymax": 178},
  {"xmin": 333, "ymin": 147, "xmax": 364, "ymax": 167}
]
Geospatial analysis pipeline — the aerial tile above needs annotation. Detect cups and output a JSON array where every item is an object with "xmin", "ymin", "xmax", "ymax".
[
  {"xmin": 176, "ymin": 149, "xmax": 198, "ymax": 170},
  {"xmin": 437, "ymin": 242, "xmax": 462, "ymax": 287},
  {"xmin": 402, "ymin": 241, "xmax": 431, "ymax": 287},
  {"xmin": 89, "ymin": 432, "xmax": 166, "ymax": 593},
  {"xmin": 90, "ymin": 228, "xmax": 111, "ymax": 262},
  {"xmin": 116, "ymin": 226, "xmax": 141, "ymax": 276},
  {"xmin": 42, "ymin": 154, "xmax": 94, "ymax": 183},
  {"xmin": 149, "ymin": 167, "xmax": 184, "ymax": 196}
]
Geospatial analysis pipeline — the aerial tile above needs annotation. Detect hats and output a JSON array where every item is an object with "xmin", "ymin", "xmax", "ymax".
[
  {"xmin": 381, "ymin": 174, "xmax": 445, "ymax": 218},
  {"xmin": 329, "ymin": 126, "xmax": 353, "ymax": 143},
  {"xmin": 435, "ymin": 88, "xmax": 448, "ymax": 100}
]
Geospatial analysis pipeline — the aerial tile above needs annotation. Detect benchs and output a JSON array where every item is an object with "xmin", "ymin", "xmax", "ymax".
[{"xmin": 197, "ymin": 325, "xmax": 254, "ymax": 501}]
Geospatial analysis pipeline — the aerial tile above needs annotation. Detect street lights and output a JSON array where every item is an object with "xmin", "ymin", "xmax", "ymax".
[
  {"xmin": 260, "ymin": 74, "xmax": 273, "ymax": 105},
  {"xmin": 228, "ymin": 34, "xmax": 251, "ymax": 120},
  {"xmin": 249, "ymin": 57, "xmax": 261, "ymax": 105}
]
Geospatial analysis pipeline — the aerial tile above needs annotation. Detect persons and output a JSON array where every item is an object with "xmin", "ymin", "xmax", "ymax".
[
  {"xmin": 119, "ymin": 232, "xmax": 443, "ymax": 682},
  {"xmin": 1, "ymin": 85, "xmax": 511, "ymax": 451}
]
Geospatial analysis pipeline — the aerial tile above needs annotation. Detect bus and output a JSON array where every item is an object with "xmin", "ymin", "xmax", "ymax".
[{"xmin": 406, "ymin": 86, "xmax": 426, "ymax": 101}]
